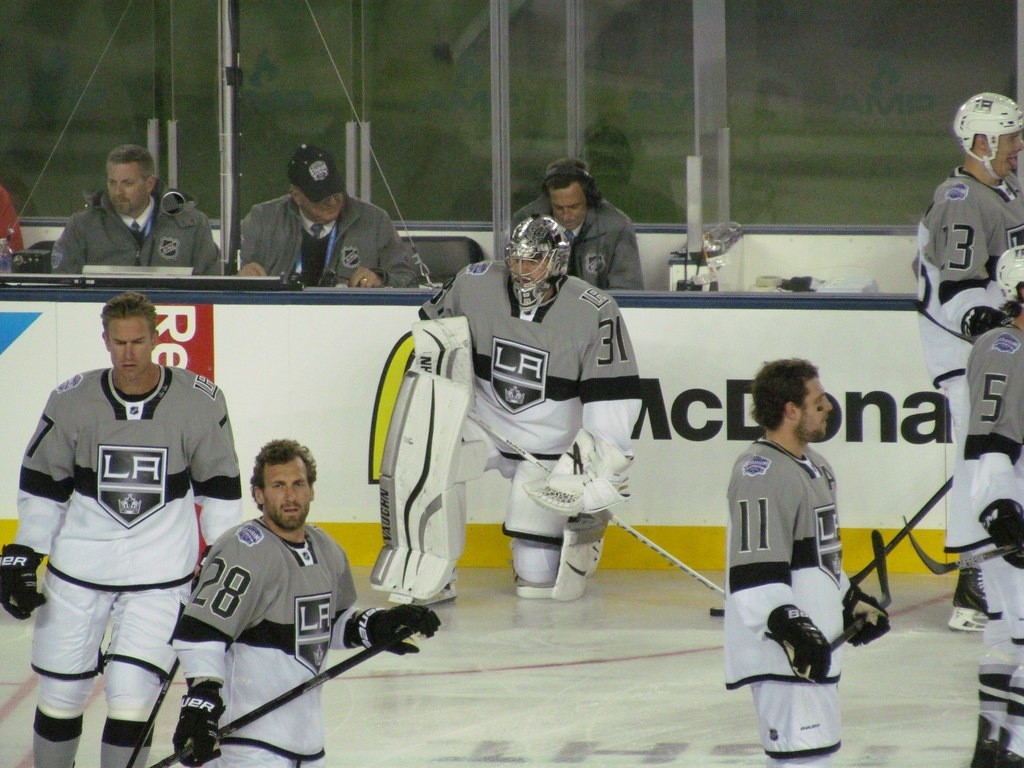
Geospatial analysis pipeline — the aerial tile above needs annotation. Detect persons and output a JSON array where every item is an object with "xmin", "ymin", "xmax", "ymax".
[
  {"xmin": 945, "ymin": 246, "xmax": 1024, "ymax": 768},
  {"xmin": 916, "ymin": 92, "xmax": 1024, "ymax": 632},
  {"xmin": 207, "ymin": 144, "xmax": 422, "ymax": 288},
  {"xmin": 0, "ymin": 186, "xmax": 24, "ymax": 255},
  {"xmin": 722, "ymin": 358, "xmax": 891, "ymax": 768},
  {"xmin": 369, "ymin": 213, "xmax": 642, "ymax": 606},
  {"xmin": 166, "ymin": 438, "xmax": 440, "ymax": 768},
  {"xmin": 511, "ymin": 157, "xmax": 643, "ymax": 290},
  {"xmin": 0, "ymin": 291, "xmax": 243, "ymax": 768},
  {"xmin": 52, "ymin": 144, "xmax": 221, "ymax": 282}
]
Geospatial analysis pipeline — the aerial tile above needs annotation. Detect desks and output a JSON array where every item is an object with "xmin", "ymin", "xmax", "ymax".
[{"xmin": 0, "ymin": 285, "xmax": 960, "ymax": 577}]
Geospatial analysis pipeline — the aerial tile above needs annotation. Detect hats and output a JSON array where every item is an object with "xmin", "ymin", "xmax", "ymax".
[{"xmin": 288, "ymin": 145, "xmax": 344, "ymax": 202}]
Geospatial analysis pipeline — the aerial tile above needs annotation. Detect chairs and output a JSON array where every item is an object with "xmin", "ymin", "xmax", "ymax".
[{"xmin": 402, "ymin": 236, "xmax": 488, "ymax": 283}]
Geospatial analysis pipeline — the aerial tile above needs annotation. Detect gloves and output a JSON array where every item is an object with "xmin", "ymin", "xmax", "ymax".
[
  {"xmin": 842, "ymin": 585, "xmax": 890, "ymax": 647},
  {"xmin": 978, "ymin": 498, "xmax": 1023, "ymax": 570},
  {"xmin": 173, "ymin": 678, "xmax": 222, "ymax": 766},
  {"xmin": 0, "ymin": 543, "xmax": 48, "ymax": 620},
  {"xmin": 960, "ymin": 306, "xmax": 1012, "ymax": 336},
  {"xmin": 763, "ymin": 604, "xmax": 831, "ymax": 682},
  {"xmin": 355, "ymin": 604, "xmax": 440, "ymax": 654}
]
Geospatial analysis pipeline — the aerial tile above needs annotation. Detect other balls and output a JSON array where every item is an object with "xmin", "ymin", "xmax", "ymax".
[{"xmin": 710, "ymin": 608, "xmax": 724, "ymax": 617}]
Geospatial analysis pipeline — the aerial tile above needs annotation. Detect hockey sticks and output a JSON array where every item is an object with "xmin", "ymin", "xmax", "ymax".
[
  {"xmin": 849, "ymin": 475, "xmax": 955, "ymax": 584},
  {"xmin": 147, "ymin": 628, "xmax": 412, "ymax": 768},
  {"xmin": 613, "ymin": 514, "xmax": 728, "ymax": 599},
  {"xmin": 126, "ymin": 655, "xmax": 180, "ymax": 768},
  {"xmin": 901, "ymin": 512, "xmax": 1018, "ymax": 576},
  {"xmin": 828, "ymin": 530, "xmax": 893, "ymax": 652}
]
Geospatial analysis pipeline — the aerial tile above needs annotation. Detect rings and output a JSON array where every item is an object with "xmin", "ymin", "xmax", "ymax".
[{"xmin": 361, "ymin": 278, "xmax": 369, "ymax": 282}]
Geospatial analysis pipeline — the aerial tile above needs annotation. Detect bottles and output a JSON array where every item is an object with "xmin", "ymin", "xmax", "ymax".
[{"xmin": 0, "ymin": 238, "xmax": 13, "ymax": 273}]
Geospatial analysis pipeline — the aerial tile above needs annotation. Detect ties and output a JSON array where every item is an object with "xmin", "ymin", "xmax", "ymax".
[
  {"xmin": 565, "ymin": 229, "xmax": 574, "ymax": 241},
  {"xmin": 311, "ymin": 224, "xmax": 322, "ymax": 241},
  {"xmin": 131, "ymin": 221, "xmax": 140, "ymax": 233}
]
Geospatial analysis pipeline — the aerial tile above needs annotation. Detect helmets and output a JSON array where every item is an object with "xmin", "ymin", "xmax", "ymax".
[
  {"xmin": 952, "ymin": 92, "xmax": 1024, "ymax": 151},
  {"xmin": 506, "ymin": 214, "xmax": 572, "ymax": 309},
  {"xmin": 995, "ymin": 244, "xmax": 1024, "ymax": 301}
]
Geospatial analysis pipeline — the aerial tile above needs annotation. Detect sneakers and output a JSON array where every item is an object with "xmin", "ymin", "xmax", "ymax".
[
  {"xmin": 972, "ymin": 715, "xmax": 1019, "ymax": 767},
  {"xmin": 948, "ymin": 567, "xmax": 996, "ymax": 631}
]
